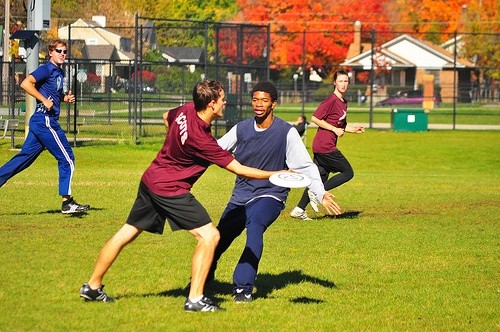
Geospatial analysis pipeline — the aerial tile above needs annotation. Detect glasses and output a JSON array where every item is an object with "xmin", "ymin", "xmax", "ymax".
[{"xmin": 56, "ymin": 48, "xmax": 67, "ymax": 54}]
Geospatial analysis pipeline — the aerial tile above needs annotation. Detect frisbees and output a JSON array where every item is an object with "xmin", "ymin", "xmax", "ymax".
[{"xmin": 269, "ymin": 171, "xmax": 312, "ymax": 188}]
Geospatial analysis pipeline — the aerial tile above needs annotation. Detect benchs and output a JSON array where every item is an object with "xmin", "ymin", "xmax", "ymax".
[{"xmin": 0, "ymin": 119, "xmax": 20, "ymax": 141}]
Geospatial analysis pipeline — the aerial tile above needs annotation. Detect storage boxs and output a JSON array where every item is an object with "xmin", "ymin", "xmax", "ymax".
[{"xmin": 391, "ymin": 109, "xmax": 429, "ymax": 132}]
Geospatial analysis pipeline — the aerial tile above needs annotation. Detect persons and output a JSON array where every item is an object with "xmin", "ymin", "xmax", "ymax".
[
  {"xmin": 292, "ymin": 115, "xmax": 310, "ymax": 137},
  {"xmin": 184, "ymin": 81, "xmax": 342, "ymax": 303},
  {"xmin": 290, "ymin": 69, "xmax": 365, "ymax": 221},
  {"xmin": 72, "ymin": 81, "xmax": 302, "ymax": 313},
  {"xmin": 0, "ymin": 39, "xmax": 91, "ymax": 214}
]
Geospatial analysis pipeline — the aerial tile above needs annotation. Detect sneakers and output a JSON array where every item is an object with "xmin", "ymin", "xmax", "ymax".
[
  {"xmin": 234, "ymin": 288, "xmax": 252, "ymax": 304},
  {"xmin": 79, "ymin": 283, "xmax": 114, "ymax": 303},
  {"xmin": 61, "ymin": 196, "xmax": 90, "ymax": 214},
  {"xmin": 307, "ymin": 189, "xmax": 318, "ymax": 213},
  {"xmin": 290, "ymin": 206, "xmax": 313, "ymax": 221},
  {"xmin": 185, "ymin": 266, "xmax": 217, "ymax": 294},
  {"xmin": 184, "ymin": 294, "xmax": 226, "ymax": 313}
]
